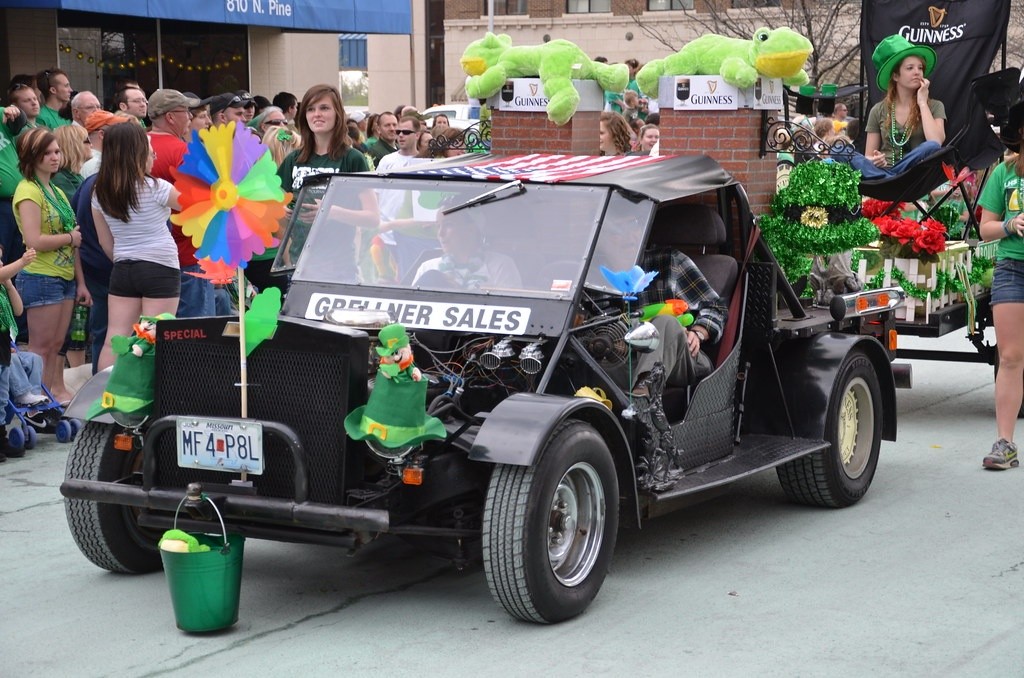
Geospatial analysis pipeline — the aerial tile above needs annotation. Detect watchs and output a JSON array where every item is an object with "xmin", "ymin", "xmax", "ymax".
[{"xmin": 689, "ymin": 329, "xmax": 705, "ymax": 344}]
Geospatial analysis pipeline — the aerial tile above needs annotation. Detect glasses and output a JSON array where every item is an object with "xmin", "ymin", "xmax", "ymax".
[
  {"xmin": 264, "ymin": 118, "xmax": 289, "ymax": 126},
  {"xmin": 10, "ymin": 83, "xmax": 27, "ymax": 96},
  {"xmin": 396, "ymin": 130, "xmax": 415, "ymax": 136},
  {"xmin": 44, "ymin": 70, "xmax": 51, "ymax": 89}
]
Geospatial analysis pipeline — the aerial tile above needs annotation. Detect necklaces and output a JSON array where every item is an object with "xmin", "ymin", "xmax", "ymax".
[
  {"xmin": 31, "ymin": 176, "xmax": 55, "ymax": 235},
  {"xmin": 887, "ymin": 97, "xmax": 914, "ymax": 166},
  {"xmin": 45, "ymin": 105, "xmax": 69, "ymax": 128},
  {"xmin": 1016, "ymin": 176, "xmax": 1024, "ymax": 213}
]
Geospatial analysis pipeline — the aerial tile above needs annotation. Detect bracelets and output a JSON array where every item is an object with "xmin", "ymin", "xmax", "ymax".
[
  {"xmin": 1001, "ymin": 217, "xmax": 1011, "ymax": 237},
  {"xmin": 68, "ymin": 232, "xmax": 74, "ymax": 245},
  {"xmin": 1010, "ymin": 218, "xmax": 1016, "ymax": 233}
]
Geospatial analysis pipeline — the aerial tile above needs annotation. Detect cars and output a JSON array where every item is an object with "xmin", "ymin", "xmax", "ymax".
[
  {"xmin": 419, "ymin": 104, "xmax": 483, "ymax": 130},
  {"xmin": 58, "ymin": 155, "xmax": 913, "ymax": 625}
]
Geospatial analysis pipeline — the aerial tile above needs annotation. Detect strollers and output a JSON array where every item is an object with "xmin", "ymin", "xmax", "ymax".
[{"xmin": 5, "ymin": 337, "xmax": 83, "ymax": 450}]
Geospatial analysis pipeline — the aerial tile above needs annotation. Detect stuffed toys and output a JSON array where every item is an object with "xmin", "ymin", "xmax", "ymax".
[
  {"xmin": 460, "ymin": 30, "xmax": 630, "ymax": 124},
  {"xmin": 374, "ymin": 322, "xmax": 422, "ymax": 384},
  {"xmin": 159, "ymin": 529, "xmax": 210, "ymax": 551},
  {"xmin": 371, "ymin": 237, "xmax": 400, "ymax": 281},
  {"xmin": 635, "ymin": 26, "xmax": 814, "ymax": 99},
  {"xmin": 111, "ymin": 313, "xmax": 176, "ymax": 357}
]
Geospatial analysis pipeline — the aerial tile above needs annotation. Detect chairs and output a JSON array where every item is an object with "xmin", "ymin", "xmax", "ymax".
[
  {"xmin": 645, "ymin": 203, "xmax": 739, "ymax": 414},
  {"xmin": 827, "ymin": 0, "xmax": 1011, "ymax": 242}
]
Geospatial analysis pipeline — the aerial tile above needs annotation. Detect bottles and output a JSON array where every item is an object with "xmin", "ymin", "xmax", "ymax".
[{"xmin": 70, "ymin": 297, "xmax": 89, "ymax": 341}]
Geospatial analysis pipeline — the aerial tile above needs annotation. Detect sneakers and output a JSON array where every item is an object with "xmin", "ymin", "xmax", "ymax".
[
  {"xmin": 983, "ymin": 438, "xmax": 1019, "ymax": 470},
  {"xmin": 22, "ymin": 407, "xmax": 64, "ymax": 434}
]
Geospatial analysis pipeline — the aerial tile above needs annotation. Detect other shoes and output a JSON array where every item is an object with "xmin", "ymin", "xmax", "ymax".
[
  {"xmin": 17, "ymin": 392, "xmax": 49, "ymax": 405},
  {"xmin": 632, "ymin": 377, "xmax": 650, "ymax": 398}
]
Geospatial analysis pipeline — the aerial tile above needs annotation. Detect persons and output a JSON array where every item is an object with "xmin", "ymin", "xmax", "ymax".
[
  {"xmin": 410, "ymin": 198, "xmax": 524, "ymax": 291},
  {"xmin": 776, "ymin": 103, "xmax": 859, "ymax": 165},
  {"xmin": 831, "ymin": 34, "xmax": 948, "ymax": 180},
  {"xmin": 977, "ymin": 95, "xmax": 1024, "ymax": 470},
  {"xmin": 901, "ymin": 113, "xmax": 1019, "ymax": 226},
  {"xmin": 0, "ymin": 67, "xmax": 491, "ymax": 465},
  {"xmin": 594, "ymin": 56, "xmax": 659, "ymax": 156},
  {"xmin": 595, "ymin": 203, "xmax": 730, "ymax": 398}
]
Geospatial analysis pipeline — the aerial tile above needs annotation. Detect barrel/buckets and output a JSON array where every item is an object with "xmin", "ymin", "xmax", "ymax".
[{"xmin": 157, "ymin": 492, "xmax": 245, "ymax": 632}]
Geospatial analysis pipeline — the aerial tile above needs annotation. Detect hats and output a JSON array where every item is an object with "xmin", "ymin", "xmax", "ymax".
[
  {"xmin": 84, "ymin": 348, "xmax": 154, "ymax": 422},
  {"xmin": 342, "ymin": 370, "xmax": 447, "ymax": 452},
  {"xmin": 350, "ymin": 110, "xmax": 366, "ymax": 123},
  {"xmin": 395, "ymin": 105, "xmax": 432, "ymax": 120},
  {"xmin": 182, "ymin": 92, "xmax": 214, "ymax": 108},
  {"xmin": 235, "ymin": 90, "xmax": 259, "ymax": 109},
  {"xmin": 253, "ymin": 96, "xmax": 272, "ymax": 114},
  {"xmin": 210, "ymin": 92, "xmax": 251, "ymax": 118},
  {"xmin": 147, "ymin": 88, "xmax": 200, "ymax": 119},
  {"xmin": 872, "ymin": 34, "xmax": 937, "ymax": 93}
]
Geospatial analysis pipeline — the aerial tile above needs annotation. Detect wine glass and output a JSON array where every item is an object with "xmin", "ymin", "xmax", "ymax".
[
  {"xmin": 754, "ymin": 78, "xmax": 763, "ymax": 106},
  {"xmin": 500, "ymin": 81, "xmax": 514, "ymax": 107},
  {"xmin": 675, "ymin": 79, "xmax": 690, "ymax": 107}
]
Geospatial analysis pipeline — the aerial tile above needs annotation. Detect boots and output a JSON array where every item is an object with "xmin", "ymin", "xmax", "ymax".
[{"xmin": 0, "ymin": 424, "xmax": 25, "ymax": 461}]
